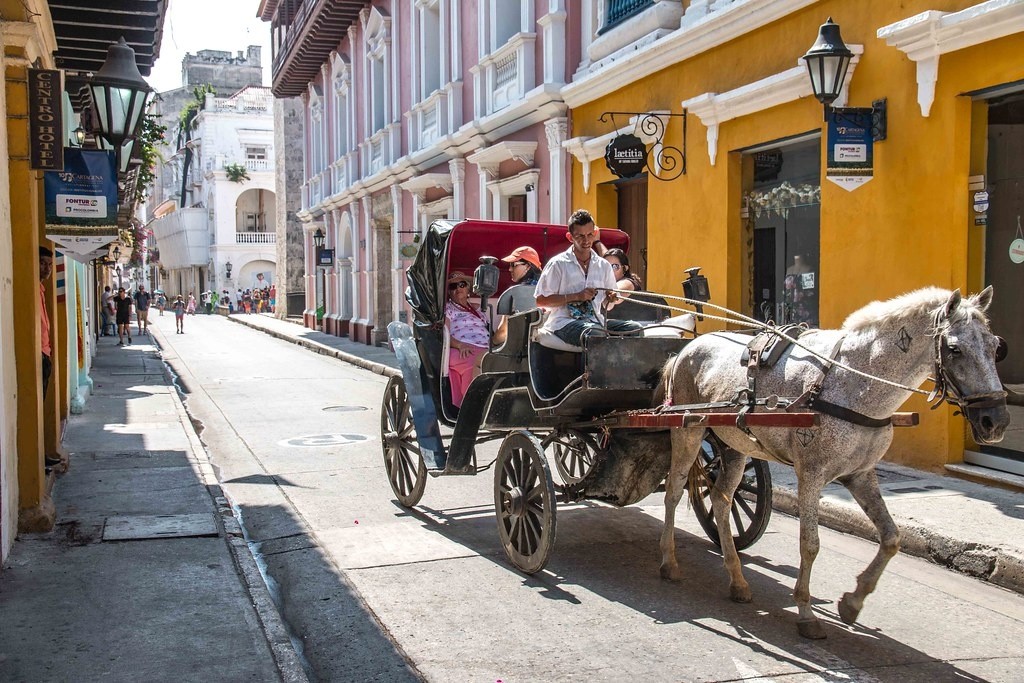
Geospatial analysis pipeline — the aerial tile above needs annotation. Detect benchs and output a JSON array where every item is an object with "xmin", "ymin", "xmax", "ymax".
[
  {"xmin": 517, "ymin": 292, "xmax": 674, "ymax": 390},
  {"xmin": 481, "ymin": 281, "xmax": 541, "ymax": 386},
  {"xmin": 441, "ymin": 297, "xmax": 508, "ymax": 419}
]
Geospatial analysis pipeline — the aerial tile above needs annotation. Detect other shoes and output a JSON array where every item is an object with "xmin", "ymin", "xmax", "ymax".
[
  {"xmin": 127, "ymin": 337, "xmax": 132, "ymax": 345},
  {"xmin": 138, "ymin": 330, "xmax": 142, "ymax": 335},
  {"xmin": 143, "ymin": 330, "xmax": 147, "ymax": 335},
  {"xmin": 44, "ymin": 466, "xmax": 52, "ymax": 474},
  {"xmin": 117, "ymin": 341, "xmax": 124, "ymax": 346},
  {"xmin": 45, "ymin": 455, "xmax": 61, "ymax": 466}
]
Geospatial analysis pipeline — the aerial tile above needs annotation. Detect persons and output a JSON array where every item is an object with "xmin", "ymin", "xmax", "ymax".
[
  {"xmin": 107, "ymin": 287, "xmax": 132, "ymax": 344},
  {"xmin": 101, "ymin": 286, "xmax": 114, "ymax": 336},
  {"xmin": 256, "ymin": 273, "xmax": 268, "ymax": 289},
  {"xmin": 221, "ymin": 290, "xmax": 233, "ymax": 314},
  {"xmin": 150, "ymin": 288, "xmax": 168, "ymax": 315},
  {"xmin": 473, "ymin": 246, "xmax": 541, "ymax": 376},
  {"xmin": 38, "ymin": 246, "xmax": 61, "ymax": 474},
  {"xmin": 236, "ymin": 284, "xmax": 276, "ymax": 315},
  {"xmin": 445, "ymin": 272, "xmax": 492, "ymax": 410},
  {"xmin": 534, "ymin": 209, "xmax": 643, "ymax": 388},
  {"xmin": 594, "ymin": 227, "xmax": 642, "ymax": 303},
  {"xmin": 186, "ymin": 292, "xmax": 195, "ymax": 315},
  {"xmin": 173, "ymin": 295, "xmax": 185, "ymax": 333},
  {"xmin": 202, "ymin": 290, "xmax": 219, "ymax": 315},
  {"xmin": 134, "ymin": 285, "xmax": 150, "ymax": 335}
]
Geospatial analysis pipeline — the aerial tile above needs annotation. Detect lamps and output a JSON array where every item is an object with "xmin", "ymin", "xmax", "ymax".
[
  {"xmin": 38, "ymin": 32, "xmax": 156, "ymax": 174},
  {"xmin": 800, "ymin": 17, "xmax": 892, "ymax": 141},
  {"xmin": 69, "ymin": 121, "xmax": 89, "ymax": 149},
  {"xmin": 112, "ymin": 266, "xmax": 121, "ymax": 278},
  {"xmin": 225, "ymin": 261, "xmax": 233, "ymax": 279},
  {"xmin": 107, "ymin": 245, "xmax": 122, "ymax": 264},
  {"xmin": 313, "ymin": 227, "xmax": 336, "ymax": 258}
]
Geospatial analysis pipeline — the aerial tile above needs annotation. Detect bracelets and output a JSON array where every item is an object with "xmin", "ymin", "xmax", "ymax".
[{"xmin": 594, "ymin": 241, "xmax": 600, "ymax": 244}]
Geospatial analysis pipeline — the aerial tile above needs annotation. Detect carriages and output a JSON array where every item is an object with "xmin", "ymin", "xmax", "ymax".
[{"xmin": 378, "ymin": 219, "xmax": 1009, "ymax": 642}]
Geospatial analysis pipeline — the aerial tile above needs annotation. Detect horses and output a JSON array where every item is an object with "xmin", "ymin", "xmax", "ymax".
[{"xmin": 651, "ymin": 285, "xmax": 1014, "ymax": 640}]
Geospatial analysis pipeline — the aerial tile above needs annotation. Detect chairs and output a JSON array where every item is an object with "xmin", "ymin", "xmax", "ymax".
[{"xmin": 100, "ymin": 311, "xmax": 117, "ymax": 337}]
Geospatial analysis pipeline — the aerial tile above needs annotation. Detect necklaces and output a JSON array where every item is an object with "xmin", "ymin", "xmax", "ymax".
[{"xmin": 578, "ymin": 257, "xmax": 590, "ymax": 268}]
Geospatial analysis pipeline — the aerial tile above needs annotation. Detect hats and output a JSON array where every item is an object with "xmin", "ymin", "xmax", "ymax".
[
  {"xmin": 448, "ymin": 271, "xmax": 471, "ymax": 286},
  {"xmin": 501, "ymin": 246, "xmax": 542, "ymax": 270}
]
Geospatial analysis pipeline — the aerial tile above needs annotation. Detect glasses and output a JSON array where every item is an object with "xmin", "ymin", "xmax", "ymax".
[
  {"xmin": 119, "ymin": 291, "xmax": 123, "ymax": 293},
  {"xmin": 448, "ymin": 281, "xmax": 468, "ymax": 291},
  {"xmin": 509, "ymin": 260, "xmax": 526, "ymax": 267},
  {"xmin": 611, "ymin": 264, "xmax": 625, "ymax": 271}
]
